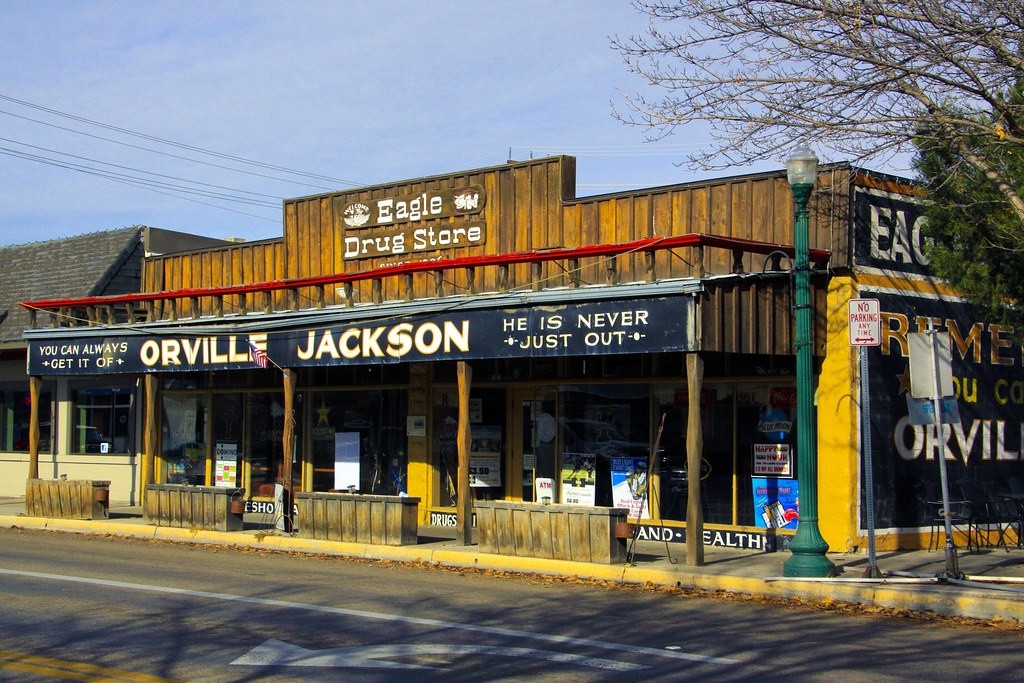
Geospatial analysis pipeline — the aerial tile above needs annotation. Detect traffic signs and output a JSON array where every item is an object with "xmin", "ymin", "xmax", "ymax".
[{"xmin": 847, "ymin": 297, "xmax": 882, "ymax": 348}]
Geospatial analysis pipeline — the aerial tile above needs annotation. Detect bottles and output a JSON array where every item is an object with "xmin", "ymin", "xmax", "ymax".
[{"xmin": 165, "ymin": 458, "xmax": 206, "ymax": 478}]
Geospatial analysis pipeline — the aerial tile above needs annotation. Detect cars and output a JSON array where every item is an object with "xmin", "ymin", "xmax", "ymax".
[{"xmin": 558, "ymin": 417, "xmax": 688, "ymax": 502}]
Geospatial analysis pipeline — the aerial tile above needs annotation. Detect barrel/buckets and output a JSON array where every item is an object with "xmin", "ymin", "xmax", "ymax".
[
  {"xmin": 615, "ymin": 511, "xmax": 636, "ymax": 538},
  {"xmin": 230, "ymin": 490, "xmax": 245, "ymax": 514}
]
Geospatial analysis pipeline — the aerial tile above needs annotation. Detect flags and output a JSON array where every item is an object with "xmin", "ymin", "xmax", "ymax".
[{"xmin": 247, "ymin": 342, "xmax": 268, "ymax": 369}]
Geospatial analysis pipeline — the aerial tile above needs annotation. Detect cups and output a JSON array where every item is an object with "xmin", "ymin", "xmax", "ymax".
[{"xmin": 399, "ymin": 491, "xmax": 408, "ymax": 497}]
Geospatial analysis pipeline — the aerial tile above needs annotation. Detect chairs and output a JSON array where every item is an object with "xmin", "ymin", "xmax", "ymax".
[
  {"xmin": 925, "ymin": 481, "xmax": 981, "ymax": 554},
  {"xmin": 984, "ymin": 479, "xmax": 1024, "ymax": 553}
]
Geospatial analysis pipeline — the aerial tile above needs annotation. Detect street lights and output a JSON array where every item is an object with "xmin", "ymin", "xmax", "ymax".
[{"xmin": 782, "ymin": 139, "xmax": 838, "ymax": 580}]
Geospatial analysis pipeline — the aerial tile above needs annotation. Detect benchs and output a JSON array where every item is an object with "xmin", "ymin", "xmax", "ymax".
[
  {"xmin": 294, "ymin": 492, "xmax": 422, "ymax": 546},
  {"xmin": 474, "ymin": 500, "xmax": 629, "ymax": 565},
  {"xmin": 25, "ymin": 479, "xmax": 111, "ymax": 520},
  {"xmin": 146, "ymin": 484, "xmax": 246, "ymax": 532}
]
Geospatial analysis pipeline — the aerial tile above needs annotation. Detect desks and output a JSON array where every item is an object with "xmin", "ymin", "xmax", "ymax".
[{"xmin": 996, "ymin": 494, "xmax": 1024, "ymax": 546}]
